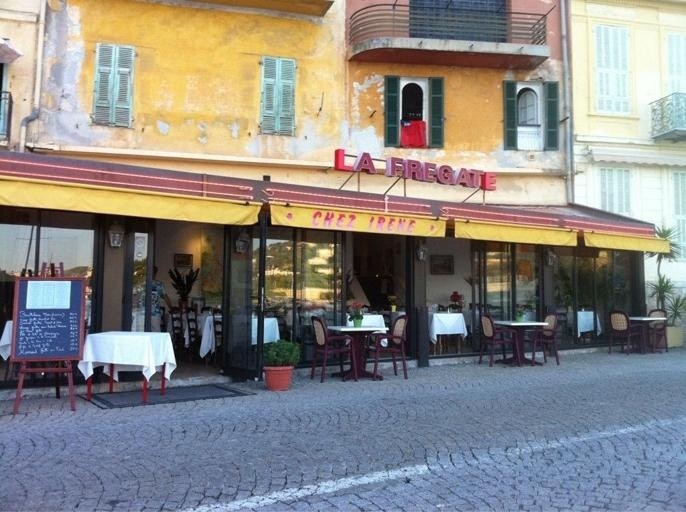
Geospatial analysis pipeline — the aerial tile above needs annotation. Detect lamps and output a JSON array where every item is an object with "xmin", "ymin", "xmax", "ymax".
[
  {"xmin": 108, "ymin": 220, "xmax": 125, "ymax": 248},
  {"xmin": 234, "ymin": 230, "xmax": 251, "ymax": 255}
]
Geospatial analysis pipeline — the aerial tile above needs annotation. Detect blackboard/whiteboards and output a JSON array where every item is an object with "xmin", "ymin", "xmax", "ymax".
[{"xmin": 11, "ymin": 277, "xmax": 86, "ymax": 362}]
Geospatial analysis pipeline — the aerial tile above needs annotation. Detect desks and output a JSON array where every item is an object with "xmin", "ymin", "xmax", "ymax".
[
  {"xmin": 80, "ymin": 331, "xmax": 167, "ymax": 404},
  {"xmin": 627, "ymin": 316, "xmax": 668, "ymax": 353}
]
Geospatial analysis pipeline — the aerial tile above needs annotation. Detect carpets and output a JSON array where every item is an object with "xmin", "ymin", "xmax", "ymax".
[{"xmin": 80, "ymin": 384, "xmax": 255, "ymax": 412}]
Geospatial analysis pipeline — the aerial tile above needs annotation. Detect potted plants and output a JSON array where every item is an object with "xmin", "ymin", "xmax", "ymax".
[
  {"xmin": 643, "ymin": 224, "xmax": 686, "ymax": 348},
  {"xmin": 254, "ymin": 338, "xmax": 297, "ymax": 391}
]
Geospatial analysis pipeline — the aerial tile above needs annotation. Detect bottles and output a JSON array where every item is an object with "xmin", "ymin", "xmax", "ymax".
[{"xmin": 432, "ymin": 304, "xmax": 438, "ymax": 312}]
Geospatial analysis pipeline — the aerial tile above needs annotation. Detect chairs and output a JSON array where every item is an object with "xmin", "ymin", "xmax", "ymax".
[
  {"xmin": 523, "ymin": 313, "xmax": 560, "ymax": 366},
  {"xmin": 428, "ymin": 303, "xmax": 468, "ymax": 356},
  {"xmin": 469, "ymin": 303, "xmax": 536, "ymax": 333},
  {"xmin": 280, "ymin": 306, "xmax": 406, "ymax": 327},
  {"xmin": 608, "ymin": 310, "xmax": 646, "ymax": 355},
  {"xmin": 480, "ymin": 315, "xmax": 521, "ymax": 368},
  {"xmin": 640, "ymin": 309, "xmax": 669, "ymax": 354},
  {"xmin": 548, "ymin": 306, "xmax": 602, "ymax": 344},
  {"xmin": 311, "ymin": 316, "xmax": 359, "ymax": 382},
  {"xmin": 161, "ymin": 303, "xmax": 225, "ymax": 367},
  {"xmin": 364, "ymin": 315, "xmax": 409, "ymax": 381}
]
